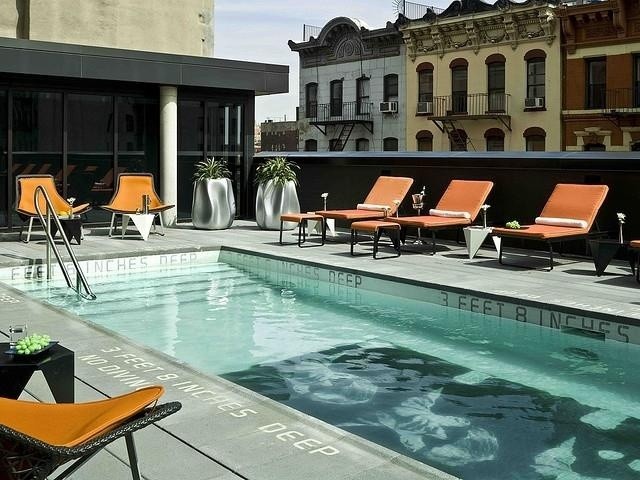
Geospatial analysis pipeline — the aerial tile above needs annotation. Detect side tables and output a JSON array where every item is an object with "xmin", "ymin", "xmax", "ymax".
[
  {"xmin": 49, "ymin": 217, "xmax": 83, "ymax": 245},
  {"xmin": 0, "ymin": 341, "xmax": 76, "ymax": 404},
  {"xmin": 306, "ymin": 211, "xmax": 335, "ymax": 238},
  {"xmin": 122, "ymin": 213, "xmax": 155, "ymax": 243},
  {"xmin": 587, "ymin": 239, "xmax": 640, "ymax": 279}
]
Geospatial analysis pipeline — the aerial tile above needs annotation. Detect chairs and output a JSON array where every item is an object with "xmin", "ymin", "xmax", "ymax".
[
  {"xmin": 0, "ymin": 385, "xmax": 183, "ymax": 480},
  {"xmin": 315, "ymin": 175, "xmax": 609, "ymax": 271},
  {"xmin": 13, "ymin": 173, "xmax": 96, "ymax": 244},
  {"xmin": 0, "ymin": 164, "xmax": 128, "ymax": 189},
  {"xmin": 98, "ymin": 172, "xmax": 175, "ymax": 236}
]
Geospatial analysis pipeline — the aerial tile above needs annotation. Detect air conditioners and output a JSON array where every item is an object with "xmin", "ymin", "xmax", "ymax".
[
  {"xmin": 524, "ymin": 98, "xmax": 544, "ymax": 109},
  {"xmin": 378, "ymin": 101, "xmax": 398, "ymax": 113},
  {"xmin": 416, "ymin": 101, "xmax": 432, "ymax": 115}
]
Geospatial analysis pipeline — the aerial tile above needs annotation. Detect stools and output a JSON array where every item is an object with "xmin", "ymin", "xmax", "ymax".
[{"xmin": 280, "ymin": 215, "xmax": 326, "ymax": 248}]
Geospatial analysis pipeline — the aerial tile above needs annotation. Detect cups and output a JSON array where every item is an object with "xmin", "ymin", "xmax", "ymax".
[{"xmin": 9, "ymin": 321, "xmax": 28, "ymax": 350}]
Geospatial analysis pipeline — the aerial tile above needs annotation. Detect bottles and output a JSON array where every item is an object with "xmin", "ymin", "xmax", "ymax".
[{"xmin": 142, "ymin": 195, "xmax": 150, "ymax": 214}]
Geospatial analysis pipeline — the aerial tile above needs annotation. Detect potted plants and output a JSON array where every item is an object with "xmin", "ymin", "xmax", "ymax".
[
  {"xmin": 191, "ymin": 156, "xmax": 237, "ymax": 230},
  {"xmin": 253, "ymin": 156, "xmax": 302, "ymax": 231}
]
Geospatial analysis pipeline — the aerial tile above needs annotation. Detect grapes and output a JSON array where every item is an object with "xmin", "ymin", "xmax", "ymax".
[
  {"xmin": 507, "ymin": 221, "xmax": 520, "ymax": 228},
  {"xmin": 16, "ymin": 335, "xmax": 51, "ymax": 354}
]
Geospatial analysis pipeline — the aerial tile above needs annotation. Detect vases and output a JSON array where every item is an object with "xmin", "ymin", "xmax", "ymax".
[{"xmin": 619, "ymin": 223, "xmax": 624, "ymax": 244}]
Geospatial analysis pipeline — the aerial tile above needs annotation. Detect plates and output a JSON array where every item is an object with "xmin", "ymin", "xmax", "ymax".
[{"xmin": 3, "ymin": 341, "xmax": 60, "ymax": 358}]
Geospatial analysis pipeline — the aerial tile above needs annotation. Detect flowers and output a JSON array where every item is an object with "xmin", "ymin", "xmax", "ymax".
[{"xmin": 617, "ymin": 212, "xmax": 626, "ymax": 224}]
